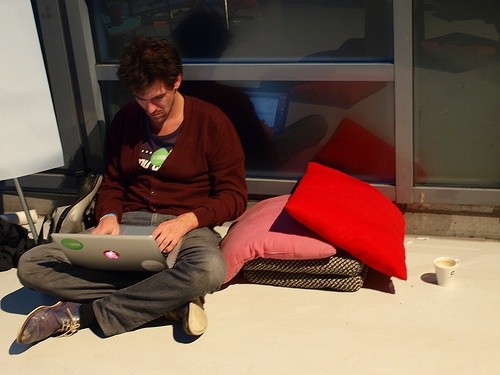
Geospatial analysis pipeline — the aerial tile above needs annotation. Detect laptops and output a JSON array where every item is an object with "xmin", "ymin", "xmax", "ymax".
[
  {"xmin": 50, "ymin": 233, "xmax": 183, "ymax": 273},
  {"xmin": 239, "ymin": 87, "xmax": 292, "ymax": 132}
]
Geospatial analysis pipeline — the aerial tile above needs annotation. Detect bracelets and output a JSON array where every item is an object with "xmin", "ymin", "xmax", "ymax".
[{"xmin": 100, "ymin": 214, "xmax": 117, "ymax": 221}]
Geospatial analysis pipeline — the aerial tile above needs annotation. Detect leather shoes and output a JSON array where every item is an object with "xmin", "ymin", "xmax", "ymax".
[
  {"xmin": 17, "ymin": 299, "xmax": 87, "ymax": 345},
  {"xmin": 164, "ymin": 295, "xmax": 208, "ymax": 336}
]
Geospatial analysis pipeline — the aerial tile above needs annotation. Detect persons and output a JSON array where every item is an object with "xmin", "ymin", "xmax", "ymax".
[
  {"xmin": 174, "ymin": 12, "xmax": 328, "ymax": 173},
  {"xmin": 16, "ymin": 38, "xmax": 248, "ymax": 344}
]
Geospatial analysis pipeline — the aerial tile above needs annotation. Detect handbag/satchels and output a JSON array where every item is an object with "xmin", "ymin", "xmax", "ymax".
[
  {"xmin": 37, "ymin": 171, "xmax": 103, "ymax": 244},
  {"xmin": 0, "ymin": 218, "xmax": 37, "ymax": 272}
]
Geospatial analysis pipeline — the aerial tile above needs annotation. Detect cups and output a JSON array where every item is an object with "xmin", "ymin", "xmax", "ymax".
[{"xmin": 434, "ymin": 257, "xmax": 460, "ymax": 287}]
